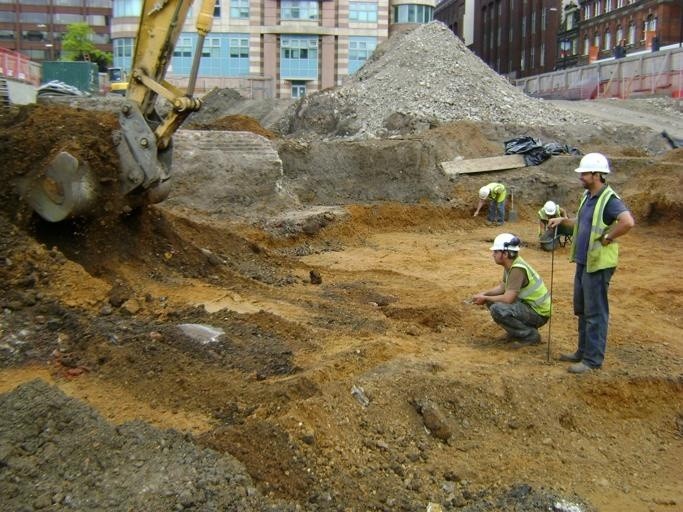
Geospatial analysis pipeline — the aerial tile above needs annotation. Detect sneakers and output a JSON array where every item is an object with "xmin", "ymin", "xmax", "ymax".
[
  {"xmin": 497, "ymin": 219, "xmax": 504, "ymax": 225},
  {"xmin": 566, "ymin": 361, "xmax": 592, "ymax": 372},
  {"xmin": 509, "ymin": 334, "xmax": 541, "ymax": 348},
  {"xmin": 487, "ymin": 220, "xmax": 493, "ymax": 225},
  {"xmin": 560, "ymin": 350, "xmax": 582, "ymax": 361}
]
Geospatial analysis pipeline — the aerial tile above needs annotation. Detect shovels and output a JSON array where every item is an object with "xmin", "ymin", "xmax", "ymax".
[{"xmin": 508, "ymin": 186, "xmax": 517, "ymax": 222}]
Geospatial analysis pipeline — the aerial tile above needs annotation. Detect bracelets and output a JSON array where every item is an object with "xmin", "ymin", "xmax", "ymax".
[{"xmin": 604, "ymin": 234, "xmax": 613, "ymax": 242}]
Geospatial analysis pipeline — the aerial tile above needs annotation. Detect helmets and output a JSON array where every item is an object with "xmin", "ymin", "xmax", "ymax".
[
  {"xmin": 488, "ymin": 233, "xmax": 520, "ymax": 252},
  {"xmin": 544, "ymin": 200, "xmax": 557, "ymax": 215},
  {"xmin": 574, "ymin": 152, "xmax": 610, "ymax": 175},
  {"xmin": 478, "ymin": 187, "xmax": 491, "ymax": 199}
]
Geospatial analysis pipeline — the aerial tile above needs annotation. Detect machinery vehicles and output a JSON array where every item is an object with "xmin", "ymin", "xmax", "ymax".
[
  {"xmin": 106, "ymin": 67, "xmax": 131, "ymax": 98},
  {"xmin": 2, "ymin": 2, "xmax": 222, "ymax": 229}
]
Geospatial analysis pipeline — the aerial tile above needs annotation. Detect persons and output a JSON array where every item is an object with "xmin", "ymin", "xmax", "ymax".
[
  {"xmin": 468, "ymin": 233, "xmax": 553, "ymax": 352},
  {"xmin": 471, "ymin": 182, "xmax": 507, "ymax": 227},
  {"xmin": 547, "ymin": 152, "xmax": 635, "ymax": 373},
  {"xmin": 535, "ymin": 199, "xmax": 568, "ymax": 253}
]
